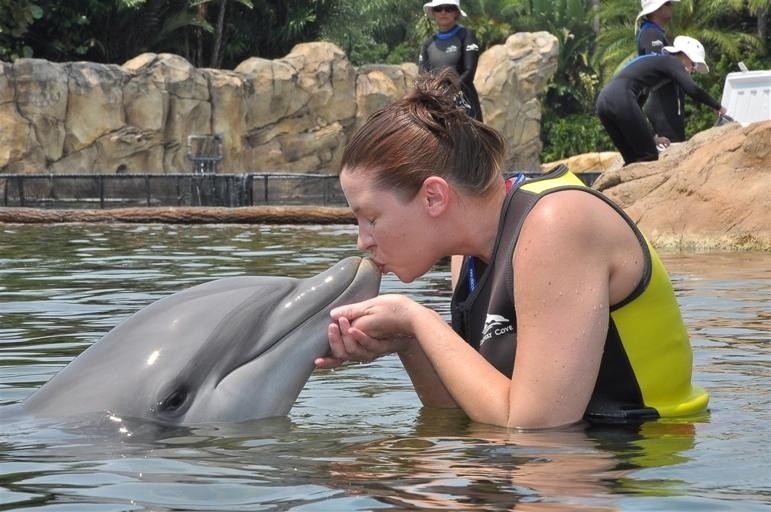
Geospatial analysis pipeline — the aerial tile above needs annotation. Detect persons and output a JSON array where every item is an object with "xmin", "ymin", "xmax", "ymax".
[
  {"xmin": 315, "ymin": 66, "xmax": 715, "ymax": 431},
  {"xmin": 326, "ymin": 410, "xmax": 698, "ymax": 512},
  {"xmin": 412, "ymin": 1, "xmax": 489, "ymax": 127},
  {"xmin": 636, "ymin": 1, "xmax": 688, "ymax": 143},
  {"xmin": 594, "ymin": 34, "xmax": 730, "ymax": 168}
]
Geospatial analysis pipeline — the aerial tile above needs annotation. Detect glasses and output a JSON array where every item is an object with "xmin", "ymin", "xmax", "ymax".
[
  {"xmin": 664, "ymin": 2, "xmax": 671, "ymax": 5},
  {"xmin": 690, "ymin": 62, "xmax": 698, "ymax": 75},
  {"xmin": 431, "ymin": 5, "xmax": 457, "ymax": 12}
]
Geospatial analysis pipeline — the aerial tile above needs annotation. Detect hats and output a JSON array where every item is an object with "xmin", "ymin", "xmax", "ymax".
[
  {"xmin": 637, "ymin": 0, "xmax": 680, "ymax": 22},
  {"xmin": 662, "ymin": 35, "xmax": 709, "ymax": 74},
  {"xmin": 423, "ymin": 0, "xmax": 468, "ymax": 20}
]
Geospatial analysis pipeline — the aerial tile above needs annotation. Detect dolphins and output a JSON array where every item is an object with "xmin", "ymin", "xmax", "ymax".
[{"xmin": 0, "ymin": 255, "xmax": 383, "ymax": 442}]
